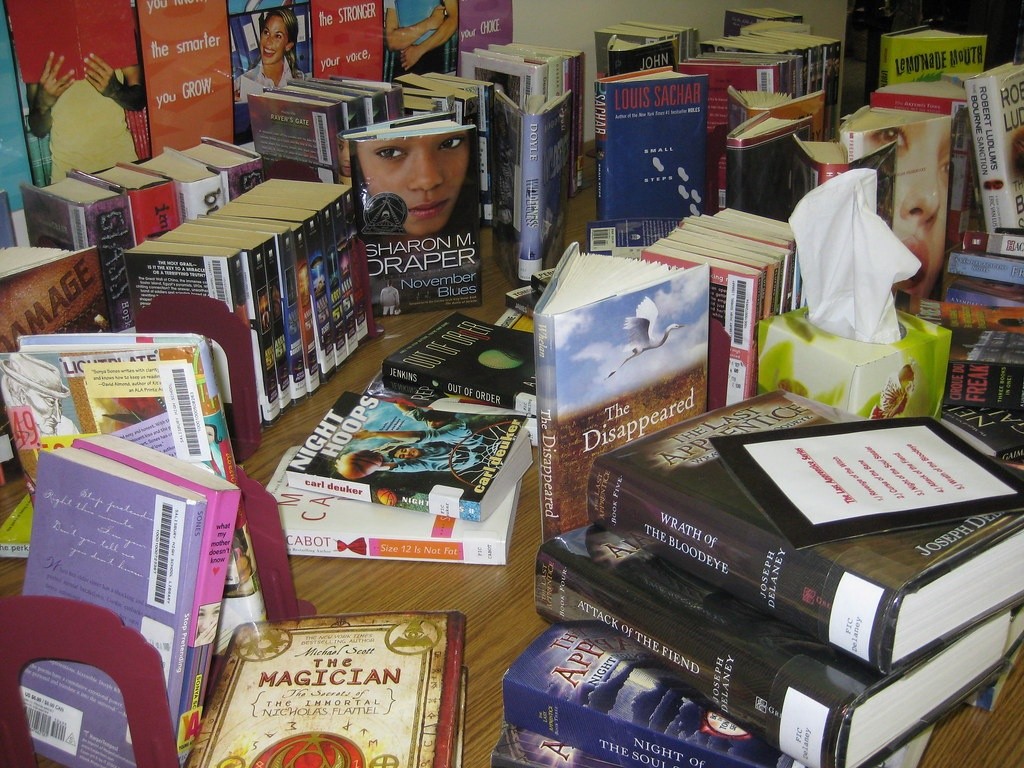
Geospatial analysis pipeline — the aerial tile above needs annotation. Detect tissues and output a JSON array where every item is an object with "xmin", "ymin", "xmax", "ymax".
[{"xmin": 755, "ymin": 167, "xmax": 952, "ymax": 423}]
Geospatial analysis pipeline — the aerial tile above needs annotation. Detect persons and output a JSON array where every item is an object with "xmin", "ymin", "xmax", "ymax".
[
  {"xmin": 864, "ymin": 115, "xmax": 951, "ymax": 313},
  {"xmin": 233, "ymin": 8, "xmax": 301, "ymax": 104},
  {"xmin": 336, "ymin": 137, "xmax": 352, "ymax": 176},
  {"xmin": 193, "ymin": 603, "xmax": 220, "ymax": 649},
  {"xmin": 27, "ymin": 50, "xmax": 146, "ymax": 185},
  {"xmin": 385, "ymin": 0, "xmax": 459, "ymax": 82},
  {"xmin": 334, "ymin": 444, "xmax": 487, "ymax": 475},
  {"xmin": 353, "ymin": 403, "xmax": 484, "ymax": 450},
  {"xmin": 356, "ymin": 129, "xmax": 471, "ymax": 236}
]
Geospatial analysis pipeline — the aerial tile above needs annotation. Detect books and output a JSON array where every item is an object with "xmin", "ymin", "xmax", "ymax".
[{"xmin": 1, "ymin": 0, "xmax": 1024, "ymax": 768}]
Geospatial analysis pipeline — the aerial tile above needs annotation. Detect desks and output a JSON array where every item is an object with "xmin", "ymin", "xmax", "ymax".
[{"xmin": 0, "ymin": 142, "xmax": 1024, "ymax": 768}]
